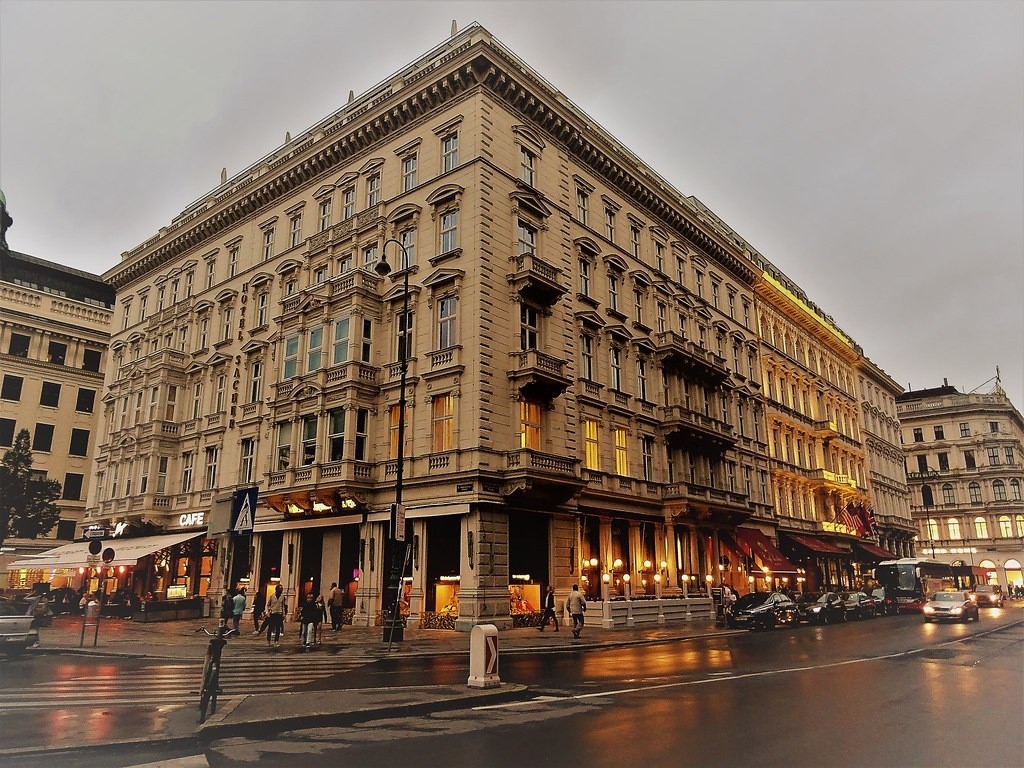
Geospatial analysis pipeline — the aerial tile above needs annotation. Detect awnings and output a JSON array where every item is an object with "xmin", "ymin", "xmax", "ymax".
[
  {"xmin": 6, "ymin": 532, "xmax": 209, "ymax": 569},
  {"xmin": 786, "ymin": 534, "xmax": 846, "ymax": 553},
  {"xmin": 858, "ymin": 543, "xmax": 898, "ymax": 558},
  {"xmin": 716, "ymin": 527, "xmax": 799, "ymax": 577}
]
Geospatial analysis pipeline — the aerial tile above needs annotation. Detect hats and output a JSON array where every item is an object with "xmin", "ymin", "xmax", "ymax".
[{"xmin": 83, "ymin": 593, "xmax": 88, "ymax": 597}]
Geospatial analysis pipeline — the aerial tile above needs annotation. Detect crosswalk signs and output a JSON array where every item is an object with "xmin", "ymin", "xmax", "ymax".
[{"xmin": 230, "ymin": 486, "xmax": 259, "ymax": 532}]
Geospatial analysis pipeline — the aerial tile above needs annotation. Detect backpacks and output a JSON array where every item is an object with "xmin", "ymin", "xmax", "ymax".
[{"xmin": 36, "ymin": 603, "xmax": 45, "ymax": 615}]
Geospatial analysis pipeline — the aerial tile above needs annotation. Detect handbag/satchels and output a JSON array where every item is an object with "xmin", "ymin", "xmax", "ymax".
[{"xmin": 262, "ymin": 610, "xmax": 271, "ymax": 621}]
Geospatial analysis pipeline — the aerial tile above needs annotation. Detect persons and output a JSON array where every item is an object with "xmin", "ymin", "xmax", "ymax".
[
  {"xmin": 50, "ymin": 587, "xmax": 160, "ymax": 619},
  {"xmin": 777, "ymin": 584, "xmax": 793, "ymax": 596},
  {"xmin": 566, "ymin": 584, "xmax": 586, "ymax": 638},
  {"xmin": 252, "ymin": 592, "xmax": 265, "ymax": 634},
  {"xmin": 723, "ymin": 584, "xmax": 736, "ymax": 613},
  {"xmin": 0, "ymin": 588, "xmax": 18, "ymax": 616},
  {"xmin": 232, "ymin": 590, "xmax": 245, "ymax": 634},
  {"xmin": 23, "ymin": 588, "xmax": 44, "ymax": 647},
  {"xmin": 1007, "ymin": 584, "xmax": 1024, "ymax": 600},
  {"xmin": 536, "ymin": 586, "xmax": 559, "ymax": 632},
  {"xmin": 221, "ymin": 595, "xmax": 232, "ymax": 632},
  {"xmin": 268, "ymin": 584, "xmax": 285, "ymax": 645},
  {"xmin": 301, "ymin": 592, "xmax": 315, "ymax": 648},
  {"xmin": 327, "ymin": 582, "xmax": 342, "ymax": 631},
  {"xmin": 315, "ymin": 595, "xmax": 327, "ymax": 644}
]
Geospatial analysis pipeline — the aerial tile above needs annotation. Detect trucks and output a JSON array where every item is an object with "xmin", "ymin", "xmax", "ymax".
[{"xmin": 0, "ymin": 615, "xmax": 42, "ymax": 660}]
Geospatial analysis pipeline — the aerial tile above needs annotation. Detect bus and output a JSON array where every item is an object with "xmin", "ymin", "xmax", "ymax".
[{"xmin": 877, "ymin": 557, "xmax": 955, "ymax": 613}]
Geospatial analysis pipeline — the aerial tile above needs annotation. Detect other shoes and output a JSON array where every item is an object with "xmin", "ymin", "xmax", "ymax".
[
  {"xmin": 81, "ymin": 614, "xmax": 85, "ymax": 616},
  {"xmin": 234, "ymin": 631, "xmax": 240, "ymax": 635},
  {"xmin": 279, "ymin": 633, "xmax": 284, "ymax": 636},
  {"xmin": 329, "ymin": 628, "xmax": 336, "ymax": 631},
  {"xmin": 537, "ymin": 628, "xmax": 544, "ymax": 632},
  {"xmin": 318, "ymin": 640, "xmax": 321, "ymax": 644},
  {"xmin": 61, "ymin": 612, "xmax": 66, "ymax": 614},
  {"xmin": 271, "ymin": 632, "xmax": 275, "ymax": 635},
  {"xmin": 25, "ymin": 647, "xmax": 30, "ymax": 649},
  {"xmin": 266, "ymin": 643, "xmax": 271, "ymax": 646},
  {"xmin": 67, "ymin": 612, "xmax": 70, "ymax": 615},
  {"xmin": 572, "ymin": 630, "xmax": 579, "ymax": 638},
  {"xmin": 32, "ymin": 644, "xmax": 39, "ymax": 647},
  {"xmin": 553, "ymin": 629, "xmax": 559, "ymax": 632},
  {"xmin": 274, "ymin": 644, "xmax": 281, "ymax": 647},
  {"xmin": 252, "ymin": 631, "xmax": 259, "ymax": 634},
  {"xmin": 312, "ymin": 641, "xmax": 317, "ymax": 645}
]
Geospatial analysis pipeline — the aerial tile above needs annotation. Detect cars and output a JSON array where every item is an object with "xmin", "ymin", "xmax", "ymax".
[
  {"xmin": 725, "ymin": 591, "xmax": 800, "ymax": 632},
  {"xmin": 975, "ymin": 583, "xmax": 1006, "ymax": 608},
  {"xmin": 839, "ymin": 591, "xmax": 876, "ymax": 621},
  {"xmin": 865, "ymin": 586, "xmax": 902, "ymax": 616},
  {"xmin": 795, "ymin": 591, "xmax": 848, "ymax": 625},
  {"xmin": 922, "ymin": 588, "xmax": 981, "ymax": 623}
]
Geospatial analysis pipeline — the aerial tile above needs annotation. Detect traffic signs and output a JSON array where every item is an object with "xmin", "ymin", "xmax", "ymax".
[{"xmin": 82, "ymin": 529, "xmax": 106, "ymax": 540}]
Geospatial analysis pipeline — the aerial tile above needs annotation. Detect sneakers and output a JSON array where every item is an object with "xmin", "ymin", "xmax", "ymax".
[
  {"xmin": 303, "ymin": 641, "xmax": 306, "ymax": 646},
  {"xmin": 306, "ymin": 643, "xmax": 311, "ymax": 647}
]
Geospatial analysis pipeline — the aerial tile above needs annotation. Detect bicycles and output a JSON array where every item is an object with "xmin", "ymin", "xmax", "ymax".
[{"xmin": 191, "ymin": 626, "xmax": 240, "ymax": 726}]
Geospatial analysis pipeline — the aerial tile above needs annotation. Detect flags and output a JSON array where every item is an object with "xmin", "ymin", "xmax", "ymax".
[{"xmin": 840, "ymin": 500, "xmax": 879, "ymax": 539}]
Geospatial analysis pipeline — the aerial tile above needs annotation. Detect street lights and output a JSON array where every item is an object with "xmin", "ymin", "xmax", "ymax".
[
  {"xmin": 375, "ymin": 239, "xmax": 406, "ymax": 643},
  {"xmin": 920, "ymin": 465, "xmax": 942, "ymax": 559}
]
